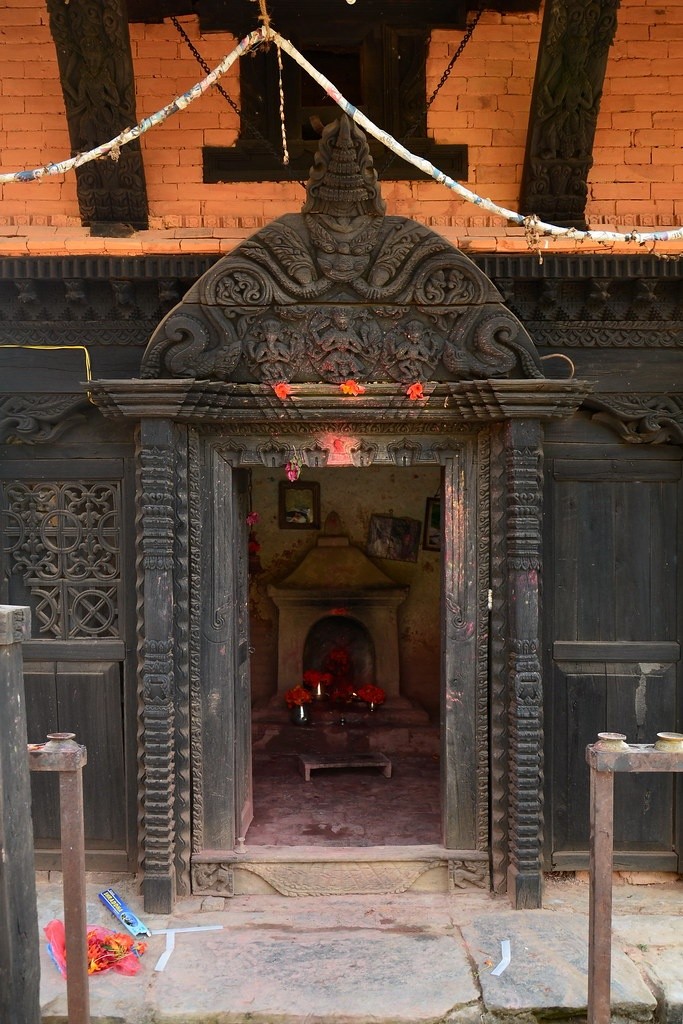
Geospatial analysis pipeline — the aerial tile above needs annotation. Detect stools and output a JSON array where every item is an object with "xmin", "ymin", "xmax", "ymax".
[{"xmin": 298, "ymin": 752, "xmax": 392, "ymax": 781}]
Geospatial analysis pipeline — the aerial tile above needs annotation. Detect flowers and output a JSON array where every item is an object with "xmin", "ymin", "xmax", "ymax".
[
  {"xmin": 247, "ymin": 513, "xmax": 260, "ymax": 553},
  {"xmin": 286, "ymin": 671, "xmax": 332, "ymax": 709},
  {"xmin": 275, "ymin": 383, "xmax": 289, "ymax": 398},
  {"xmin": 358, "ymin": 685, "xmax": 386, "ymax": 705},
  {"xmin": 407, "ymin": 381, "xmax": 423, "ymax": 401},
  {"xmin": 340, "ymin": 380, "xmax": 365, "ymax": 396},
  {"xmin": 284, "ymin": 446, "xmax": 302, "ymax": 482}
]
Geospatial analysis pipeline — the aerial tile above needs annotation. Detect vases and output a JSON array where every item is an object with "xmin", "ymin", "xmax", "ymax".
[
  {"xmin": 368, "ymin": 701, "xmax": 377, "ymax": 711},
  {"xmin": 311, "ymin": 681, "xmax": 325, "ymax": 701},
  {"xmin": 291, "ymin": 703, "xmax": 310, "ymax": 725}
]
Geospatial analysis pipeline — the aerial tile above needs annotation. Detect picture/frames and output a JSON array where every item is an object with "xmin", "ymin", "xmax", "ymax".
[
  {"xmin": 278, "ymin": 481, "xmax": 321, "ymax": 529},
  {"xmin": 423, "ymin": 497, "xmax": 441, "ymax": 552}
]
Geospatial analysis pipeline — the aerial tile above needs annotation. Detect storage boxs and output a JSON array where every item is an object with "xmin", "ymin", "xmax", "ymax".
[{"xmin": 99, "ymin": 889, "xmax": 151, "ymax": 939}]
